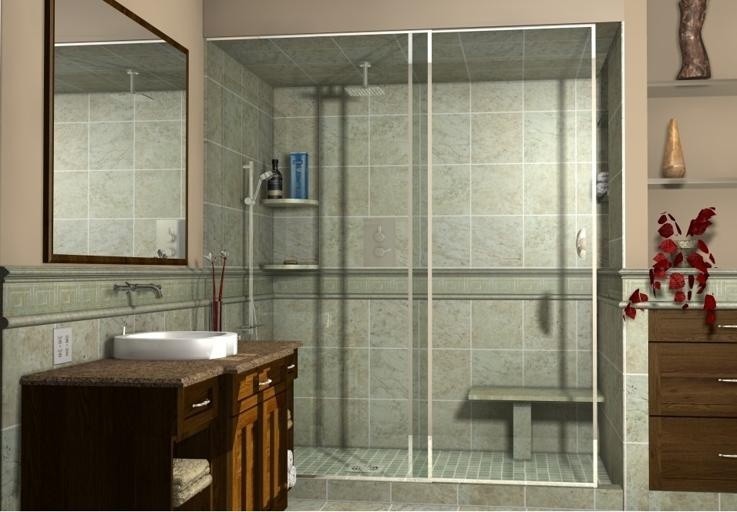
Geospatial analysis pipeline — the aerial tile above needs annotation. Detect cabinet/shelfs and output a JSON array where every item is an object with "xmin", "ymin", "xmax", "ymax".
[
  {"xmin": 20, "ymin": 377, "xmax": 226, "ymax": 511},
  {"xmin": 288, "ymin": 348, "xmax": 294, "ymax": 491},
  {"xmin": 260, "ymin": 198, "xmax": 321, "ymax": 271},
  {"xmin": 647, "ymin": 310, "xmax": 736, "ymax": 494},
  {"xmin": 221, "ymin": 354, "xmax": 288, "ymax": 511},
  {"xmin": 646, "ymin": 79, "xmax": 736, "ymax": 188}
]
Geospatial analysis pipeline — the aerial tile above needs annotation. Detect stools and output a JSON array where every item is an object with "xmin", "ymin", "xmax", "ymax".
[{"xmin": 467, "ymin": 385, "xmax": 605, "ymax": 460}]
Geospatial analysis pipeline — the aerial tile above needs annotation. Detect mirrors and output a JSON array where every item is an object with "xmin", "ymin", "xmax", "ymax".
[{"xmin": 42, "ymin": 0, "xmax": 190, "ymax": 265}]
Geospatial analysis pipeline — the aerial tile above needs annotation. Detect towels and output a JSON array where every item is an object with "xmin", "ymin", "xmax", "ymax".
[
  {"xmin": 287, "ymin": 449, "xmax": 293, "ymax": 471},
  {"xmin": 172, "ymin": 457, "xmax": 213, "ymax": 506},
  {"xmin": 288, "ymin": 466, "xmax": 296, "ymax": 487},
  {"xmin": 288, "ymin": 409, "xmax": 293, "ymax": 429}
]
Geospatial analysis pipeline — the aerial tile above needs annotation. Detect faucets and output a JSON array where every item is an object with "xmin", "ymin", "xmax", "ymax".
[{"xmin": 113, "ymin": 281, "xmax": 163, "ymax": 300}]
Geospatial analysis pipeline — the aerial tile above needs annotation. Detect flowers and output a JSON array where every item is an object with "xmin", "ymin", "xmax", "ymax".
[
  {"xmin": 624, "ymin": 207, "xmax": 719, "ymax": 326},
  {"xmin": 203, "ymin": 249, "xmax": 229, "ymax": 302}
]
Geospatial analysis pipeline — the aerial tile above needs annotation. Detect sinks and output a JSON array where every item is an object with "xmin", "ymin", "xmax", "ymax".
[{"xmin": 114, "ymin": 330, "xmax": 239, "ymax": 360}]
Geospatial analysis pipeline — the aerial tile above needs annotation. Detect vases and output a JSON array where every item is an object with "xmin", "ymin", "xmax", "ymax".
[
  {"xmin": 212, "ymin": 301, "xmax": 222, "ymax": 331},
  {"xmin": 661, "ymin": 119, "xmax": 686, "ymax": 178},
  {"xmin": 670, "ymin": 238, "xmax": 697, "ymax": 268},
  {"xmin": 676, "ymin": 0, "xmax": 712, "ymax": 80}
]
